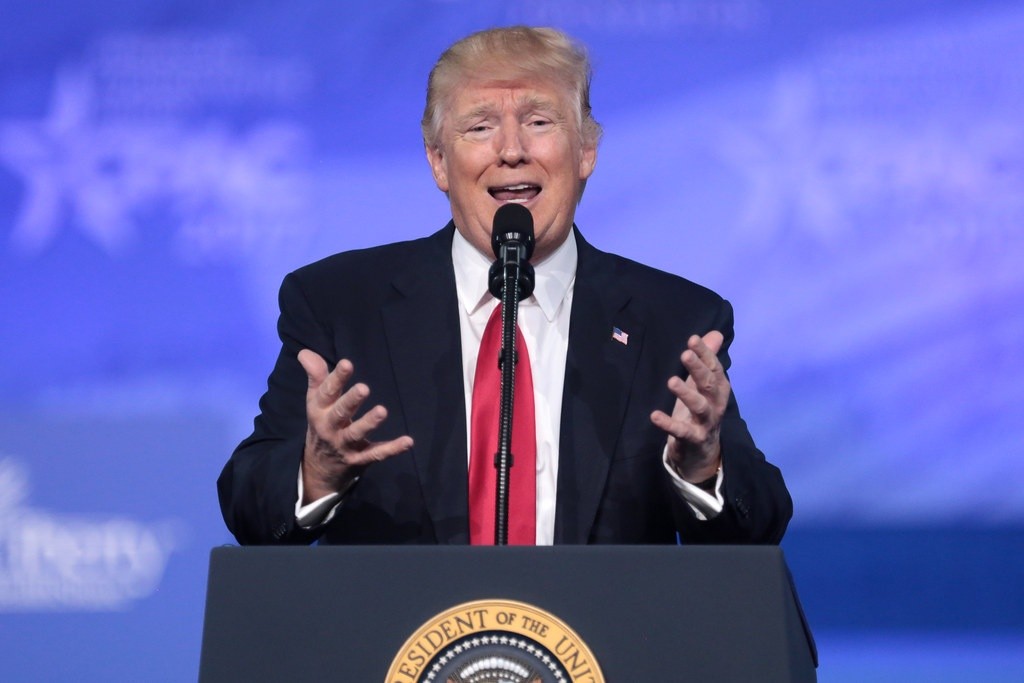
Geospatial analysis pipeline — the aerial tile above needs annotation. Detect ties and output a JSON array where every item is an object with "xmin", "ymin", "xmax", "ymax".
[{"xmin": 469, "ymin": 299, "xmax": 535, "ymax": 546}]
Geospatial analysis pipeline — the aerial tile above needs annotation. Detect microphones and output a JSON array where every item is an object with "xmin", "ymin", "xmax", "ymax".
[{"xmin": 491, "ymin": 203, "xmax": 535, "ymax": 280}]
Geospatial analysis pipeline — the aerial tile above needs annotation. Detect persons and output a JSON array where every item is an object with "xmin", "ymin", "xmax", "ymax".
[{"xmin": 218, "ymin": 26, "xmax": 792, "ymax": 545}]
